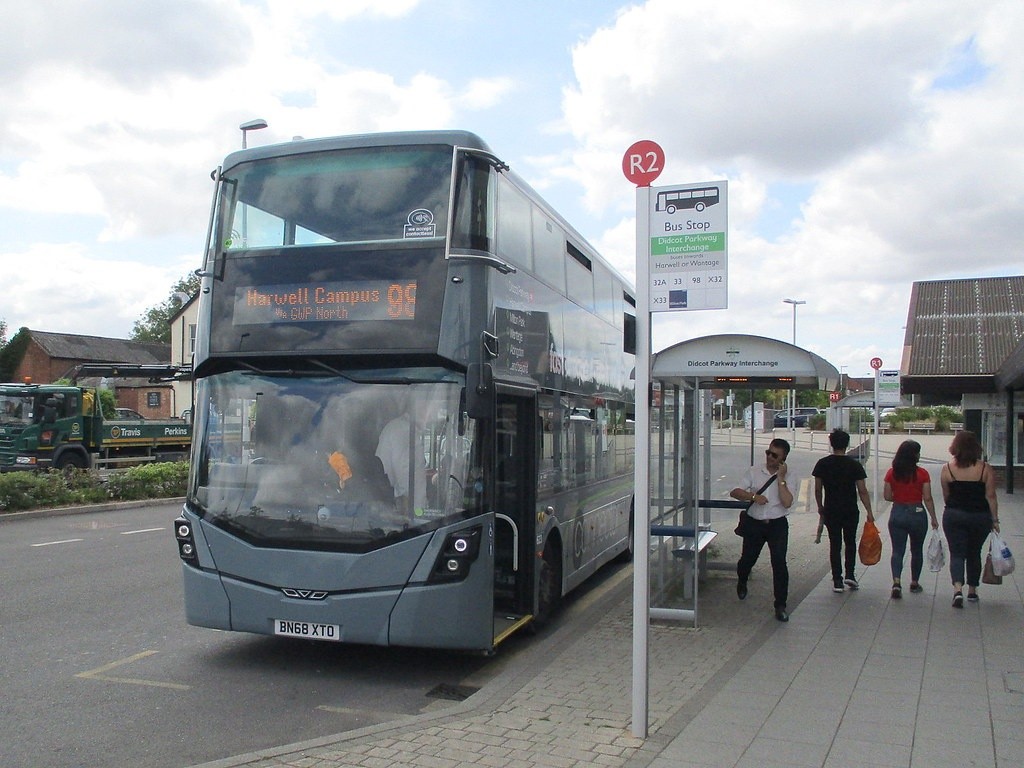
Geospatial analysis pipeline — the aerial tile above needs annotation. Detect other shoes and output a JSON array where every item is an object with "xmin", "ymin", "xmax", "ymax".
[
  {"xmin": 910, "ymin": 582, "xmax": 923, "ymax": 592},
  {"xmin": 951, "ymin": 591, "xmax": 964, "ymax": 608},
  {"xmin": 891, "ymin": 583, "xmax": 902, "ymax": 598},
  {"xmin": 967, "ymin": 594, "xmax": 979, "ymax": 602},
  {"xmin": 833, "ymin": 576, "xmax": 844, "ymax": 592},
  {"xmin": 844, "ymin": 569, "xmax": 859, "ymax": 587},
  {"xmin": 775, "ymin": 610, "xmax": 788, "ymax": 622},
  {"xmin": 737, "ymin": 576, "xmax": 748, "ymax": 599}
]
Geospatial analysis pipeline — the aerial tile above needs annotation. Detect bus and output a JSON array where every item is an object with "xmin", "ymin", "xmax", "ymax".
[{"xmin": 173, "ymin": 128, "xmax": 638, "ymax": 655}]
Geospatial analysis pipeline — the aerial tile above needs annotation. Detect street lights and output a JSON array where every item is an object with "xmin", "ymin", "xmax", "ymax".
[
  {"xmin": 840, "ymin": 365, "xmax": 849, "ymax": 396},
  {"xmin": 239, "ymin": 118, "xmax": 270, "ymax": 247},
  {"xmin": 784, "ymin": 298, "xmax": 807, "ymax": 449},
  {"xmin": 862, "ymin": 373, "xmax": 871, "ymax": 391}
]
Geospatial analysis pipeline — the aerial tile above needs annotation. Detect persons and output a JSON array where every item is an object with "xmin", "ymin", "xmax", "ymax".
[
  {"xmin": 940, "ymin": 430, "xmax": 1001, "ymax": 609},
  {"xmin": 883, "ymin": 438, "xmax": 940, "ymax": 599},
  {"xmin": 728, "ymin": 438, "xmax": 802, "ymax": 622},
  {"xmin": 375, "ymin": 387, "xmax": 468, "ymax": 520},
  {"xmin": 811, "ymin": 428, "xmax": 876, "ymax": 592}
]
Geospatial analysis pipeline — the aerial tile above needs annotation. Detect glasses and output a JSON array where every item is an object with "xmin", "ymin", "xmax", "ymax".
[{"xmin": 765, "ymin": 449, "xmax": 779, "ymax": 459}]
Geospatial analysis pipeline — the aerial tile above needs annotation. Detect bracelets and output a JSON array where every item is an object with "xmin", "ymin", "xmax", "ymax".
[
  {"xmin": 778, "ymin": 481, "xmax": 786, "ymax": 485},
  {"xmin": 993, "ymin": 519, "xmax": 1000, "ymax": 523},
  {"xmin": 751, "ymin": 494, "xmax": 756, "ymax": 500}
]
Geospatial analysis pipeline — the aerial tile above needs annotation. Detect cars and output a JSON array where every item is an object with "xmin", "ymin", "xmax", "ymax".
[
  {"xmin": 879, "ymin": 408, "xmax": 895, "ymax": 416},
  {"xmin": 774, "ymin": 408, "xmax": 821, "ymax": 428}
]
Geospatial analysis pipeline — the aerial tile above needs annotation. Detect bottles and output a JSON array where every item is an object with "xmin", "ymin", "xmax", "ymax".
[{"xmin": 317, "ymin": 506, "xmax": 331, "ymax": 521}]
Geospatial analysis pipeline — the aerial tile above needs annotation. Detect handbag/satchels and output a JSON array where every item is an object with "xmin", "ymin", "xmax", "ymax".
[
  {"xmin": 990, "ymin": 529, "xmax": 1015, "ymax": 576},
  {"xmin": 734, "ymin": 511, "xmax": 746, "ymax": 536},
  {"xmin": 980, "ymin": 554, "xmax": 1002, "ymax": 585},
  {"xmin": 925, "ymin": 528, "xmax": 945, "ymax": 572},
  {"xmin": 858, "ymin": 521, "xmax": 882, "ymax": 565}
]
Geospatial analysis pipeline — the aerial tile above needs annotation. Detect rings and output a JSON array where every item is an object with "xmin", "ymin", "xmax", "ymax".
[{"xmin": 783, "ymin": 465, "xmax": 784, "ymax": 466}]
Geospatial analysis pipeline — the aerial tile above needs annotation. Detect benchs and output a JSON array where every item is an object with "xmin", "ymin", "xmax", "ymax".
[
  {"xmin": 904, "ymin": 422, "xmax": 935, "ymax": 435},
  {"xmin": 861, "ymin": 422, "xmax": 890, "ymax": 435},
  {"xmin": 950, "ymin": 423, "xmax": 965, "ymax": 434},
  {"xmin": 672, "ymin": 530, "xmax": 718, "ymax": 599}
]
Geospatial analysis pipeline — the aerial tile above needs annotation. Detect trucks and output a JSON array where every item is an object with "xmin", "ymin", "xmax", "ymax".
[{"xmin": 0, "ymin": 364, "xmax": 193, "ymax": 473}]
